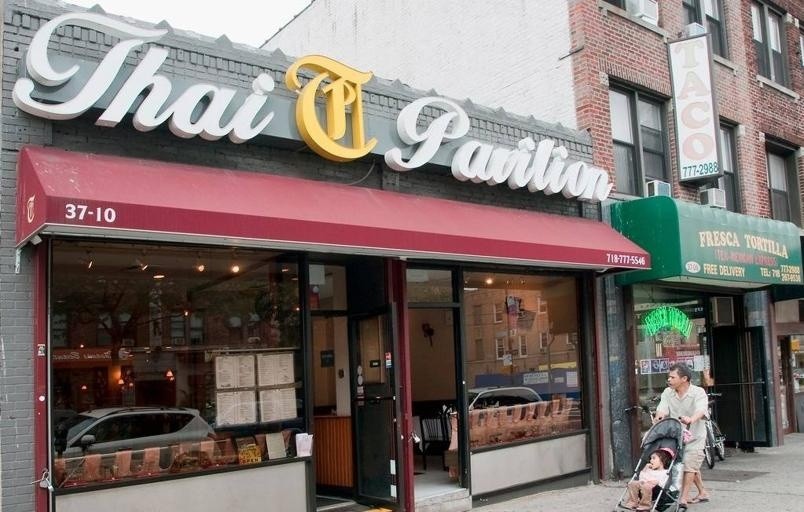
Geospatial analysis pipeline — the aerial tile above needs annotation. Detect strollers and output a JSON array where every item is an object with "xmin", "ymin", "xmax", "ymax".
[{"xmin": 611, "ymin": 417, "xmax": 690, "ymax": 511}]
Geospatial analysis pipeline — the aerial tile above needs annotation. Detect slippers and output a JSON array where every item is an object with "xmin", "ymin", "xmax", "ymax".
[
  {"xmin": 679, "ymin": 502, "xmax": 688, "ymax": 509},
  {"xmin": 688, "ymin": 497, "xmax": 709, "ymax": 504}
]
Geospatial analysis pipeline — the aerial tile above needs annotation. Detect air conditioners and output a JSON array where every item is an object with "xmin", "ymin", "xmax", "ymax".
[
  {"xmin": 644, "ymin": 180, "xmax": 671, "ymax": 197},
  {"xmin": 624, "ymin": 0, "xmax": 706, "ymax": 39},
  {"xmin": 699, "ymin": 187, "xmax": 727, "ymax": 209}
]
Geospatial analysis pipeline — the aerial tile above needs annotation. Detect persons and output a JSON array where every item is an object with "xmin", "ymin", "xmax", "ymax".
[
  {"xmin": 651, "ymin": 362, "xmax": 711, "ymax": 512},
  {"xmin": 625, "ymin": 449, "xmax": 673, "ymax": 512}
]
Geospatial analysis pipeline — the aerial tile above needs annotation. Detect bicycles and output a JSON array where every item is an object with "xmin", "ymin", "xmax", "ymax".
[
  {"xmin": 625, "ymin": 396, "xmax": 666, "ymax": 428},
  {"xmin": 702, "ymin": 393, "xmax": 729, "ymax": 468}
]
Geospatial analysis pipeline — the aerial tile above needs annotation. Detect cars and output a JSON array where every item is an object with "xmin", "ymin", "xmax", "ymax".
[
  {"xmin": 55, "ymin": 405, "xmax": 224, "ymax": 466},
  {"xmin": 456, "ymin": 384, "xmax": 543, "ymax": 420}
]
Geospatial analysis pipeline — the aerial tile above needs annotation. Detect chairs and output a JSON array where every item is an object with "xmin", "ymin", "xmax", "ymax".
[{"xmin": 418, "ymin": 403, "xmax": 455, "ymax": 471}]
